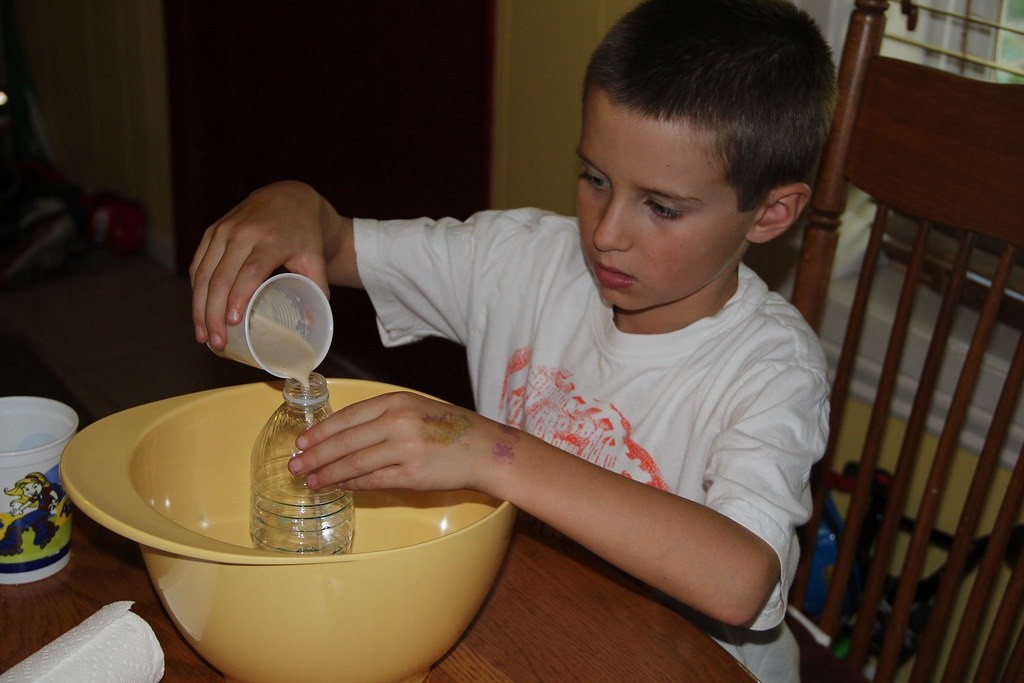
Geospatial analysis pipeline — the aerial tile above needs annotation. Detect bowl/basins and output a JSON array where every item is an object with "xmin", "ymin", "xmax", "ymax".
[{"xmin": 60, "ymin": 381, "xmax": 515, "ymax": 681}]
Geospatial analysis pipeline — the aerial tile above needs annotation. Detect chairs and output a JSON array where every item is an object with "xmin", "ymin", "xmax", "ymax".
[{"xmin": 784, "ymin": 3, "xmax": 1024, "ymax": 683}]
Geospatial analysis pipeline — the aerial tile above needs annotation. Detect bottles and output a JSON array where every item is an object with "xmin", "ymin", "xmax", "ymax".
[{"xmin": 250, "ymin": 371, "xmax": 355, "ymax": 555}]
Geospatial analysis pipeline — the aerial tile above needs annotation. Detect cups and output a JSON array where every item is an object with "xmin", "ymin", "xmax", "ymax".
[
  {"xmin": 206, "ymin": 273, "xmax": 334, "ymax": 378},
  {"xmin": 0, "ymin": 396, "xmax": 79, "ymax": 585}
]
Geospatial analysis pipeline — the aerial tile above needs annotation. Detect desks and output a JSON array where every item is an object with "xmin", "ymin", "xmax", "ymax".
[{"xmin": 0, "ymin": 254, "xmax": 762, "ymax": 683}]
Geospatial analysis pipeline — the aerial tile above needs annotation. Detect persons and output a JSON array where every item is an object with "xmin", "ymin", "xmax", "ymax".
[{"xmin": 189, "ymin": 0, "xmax": 842, "ymax": 683}]
